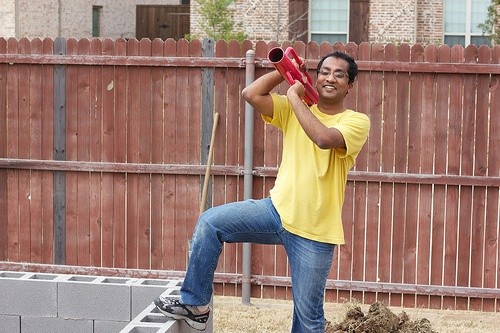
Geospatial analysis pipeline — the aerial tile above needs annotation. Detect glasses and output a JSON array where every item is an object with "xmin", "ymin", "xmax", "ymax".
[{"xmin": 317, "ymin": 69, "xmax": 350, "ymax": 80}]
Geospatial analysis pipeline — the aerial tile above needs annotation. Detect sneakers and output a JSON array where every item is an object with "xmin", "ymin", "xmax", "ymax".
[{"xmin": 153, "ymin": 297, "xmax": 211, "ymax": 331}]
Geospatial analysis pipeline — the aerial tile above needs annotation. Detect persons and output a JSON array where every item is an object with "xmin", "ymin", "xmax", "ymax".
[{"xmin": 153, "ymin": 51, "xmax": 371, "ymax": 332}]
